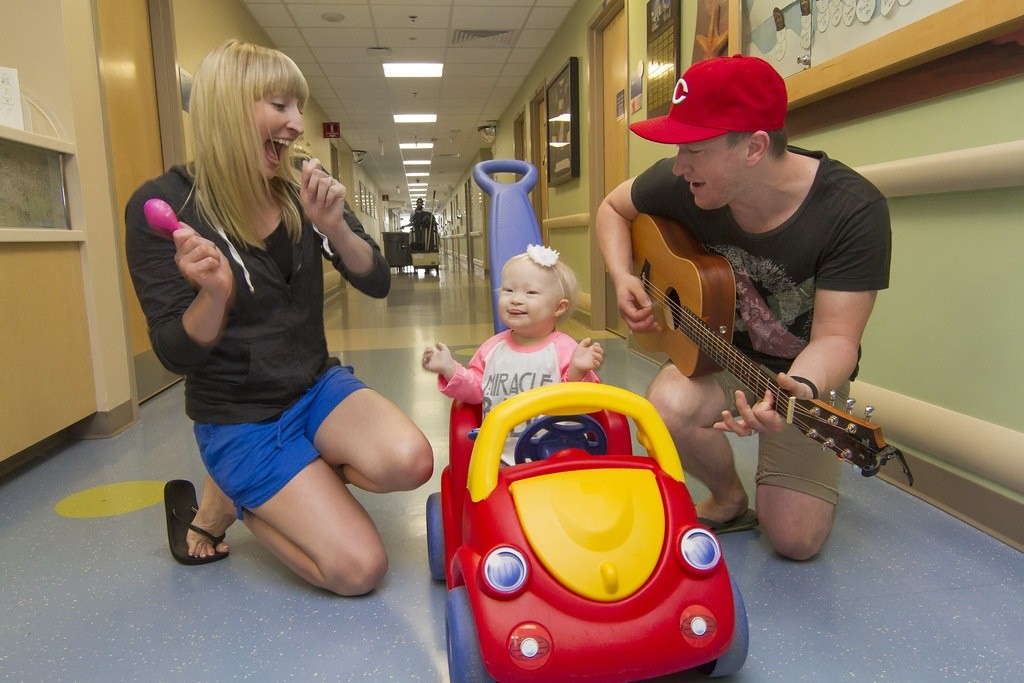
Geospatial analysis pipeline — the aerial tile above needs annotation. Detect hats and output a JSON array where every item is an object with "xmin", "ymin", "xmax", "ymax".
[{"xmin": 623, "ymin": 54, "xmax": 789, "ymax": 144}]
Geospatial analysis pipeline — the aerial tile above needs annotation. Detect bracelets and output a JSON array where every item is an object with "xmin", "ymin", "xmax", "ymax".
[{"xmin": 791, "ymin": 375, "xmax": 818, "ymax": 398}]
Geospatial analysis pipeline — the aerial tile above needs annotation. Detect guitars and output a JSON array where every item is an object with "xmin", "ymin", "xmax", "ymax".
[{"xmin": 627, "ymin": 210, "xmax": 915, "ymax": 488}]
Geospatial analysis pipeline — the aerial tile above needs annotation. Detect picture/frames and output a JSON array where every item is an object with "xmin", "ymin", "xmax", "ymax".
[
  {"xmin": 547, "ymin": 56, "xmax": 580, "ymax": 187},
  {"xmin": 646, "ymin": 0, "xmax": 681, "ymax": 122}
]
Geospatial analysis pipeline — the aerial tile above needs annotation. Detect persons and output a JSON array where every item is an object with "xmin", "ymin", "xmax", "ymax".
[
  {"xmin": 399, "ymin": 198, "xmax": 427, "ymax": 228},
  {"xmin": 124, "ymin": 38, "xmax": 433, "ymax": 596},
  {"xmin": 596, "ymin": 53, "xmax": 892, "ymax": 561},
  {"xmin": 421, "ymin": 243, "xmax": 604, "ymax": 466}
]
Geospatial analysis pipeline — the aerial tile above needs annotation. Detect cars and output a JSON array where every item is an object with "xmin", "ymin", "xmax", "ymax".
[{"xmin": 426, "ymin": 355, "xmax": 751, "ymax": 683}]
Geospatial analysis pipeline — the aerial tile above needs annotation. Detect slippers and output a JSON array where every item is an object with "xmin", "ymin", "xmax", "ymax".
[
  {"xmin": 691, "ymin": 499, "xmax": 761, "ymax": 533},
  {"xmin": 162, "ymin": 477, "xmax": 227, "ymax": 564}
]
